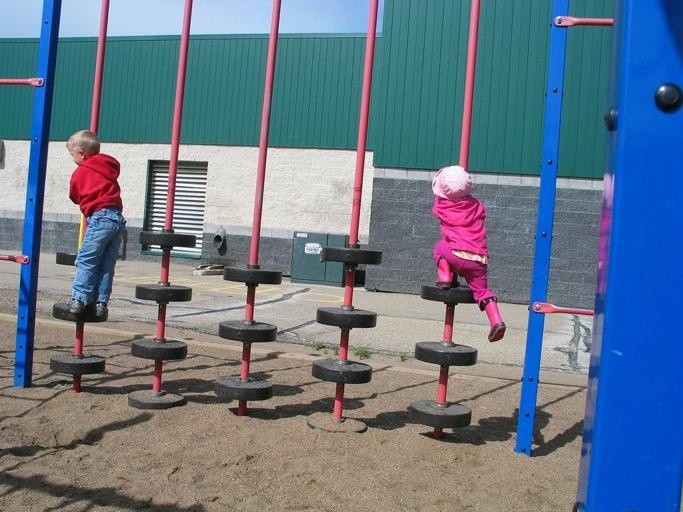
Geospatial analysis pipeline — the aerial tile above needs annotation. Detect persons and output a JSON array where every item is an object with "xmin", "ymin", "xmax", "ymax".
[
  {"xmin": 432, "ymin": 165, "xmax": 506, "ymax": 342},
  {"xmin": 65, "ymin": 129, "xmax": 127, "ymax": 318}
]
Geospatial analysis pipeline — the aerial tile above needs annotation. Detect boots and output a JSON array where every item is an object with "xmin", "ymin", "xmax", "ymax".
[
  {"xmin": 485, "ymin": 300, "xmax": 507, "ymax": 343},
  {"xmin": 435, "ymin": 258, "xmax": 458, "ymax": 288}
]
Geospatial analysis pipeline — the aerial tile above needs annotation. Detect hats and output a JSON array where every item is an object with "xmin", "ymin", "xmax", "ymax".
[{"xmin": 432, "ymin": 164, "xmax": 475, "ymax": 200}]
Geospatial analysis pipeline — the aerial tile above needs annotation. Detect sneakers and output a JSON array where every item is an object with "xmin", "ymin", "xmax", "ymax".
[{"xmin": 67, "ymin": 298, "xmax": 108, "ymax": 317}]
